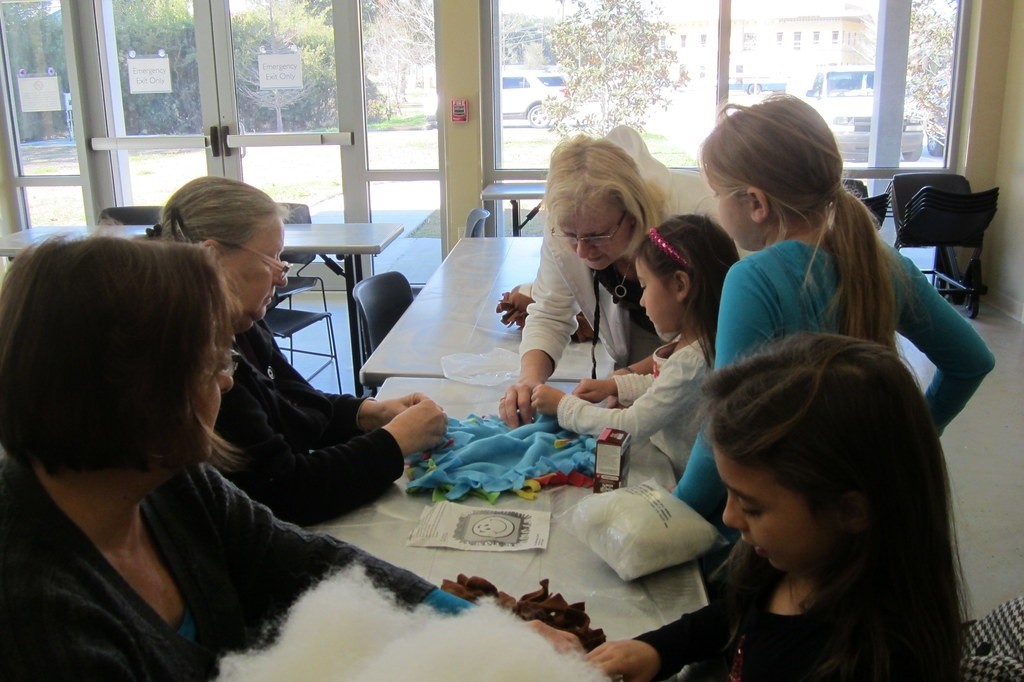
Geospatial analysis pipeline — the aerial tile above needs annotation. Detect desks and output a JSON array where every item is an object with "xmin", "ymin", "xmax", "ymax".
[
  {"xmin": 359, "ymin": 237, "xmax": 615, "ymax": 382},
  {"xmin": 0, "ymin": 223, "xmax": 404, "ymax": 398},
  {"xmin": 303, "ymin": 376, "xmax": 710, "ymax": 680},
  {"xmin": 480, "ymin": 183, "xmax": 545, "ymax": 237}
]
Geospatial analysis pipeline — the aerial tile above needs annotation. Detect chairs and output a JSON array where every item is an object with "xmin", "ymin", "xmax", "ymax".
[
  {"xmin": 98, "ymin": 206, "xmax": 163, "ymax": 225},
  {"xmin": 842, "ymin": 179, "xmax": 890, "ymax": 230},
  {"xmin": 352, "ymin": 271, "xmax": 414, "ymax": 397},
  {"xmin": 882, "ymin": 173, "xmax": 1001, "ymax": 318},
  {"xmin": 275, "ymin": 203, "xmax": 334, "ymax": 381},
  {"xmin": 265, "ymin": 307, "xmax": 342, "ymax": 395},
  {"xmin": 465, "ymin": 208, "xmax": 490, "ymax": 237}
]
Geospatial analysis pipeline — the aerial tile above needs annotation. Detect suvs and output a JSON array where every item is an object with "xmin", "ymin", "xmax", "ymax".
[
  {"xmin": 806, "ymin": 67, "xmax": 924, "ymax": 163},
  {"xmin": 427, "ymin": 71, "xmax": 574, "ymax": 127}
]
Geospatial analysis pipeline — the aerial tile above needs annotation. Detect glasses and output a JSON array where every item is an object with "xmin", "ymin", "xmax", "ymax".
[
  {"xmin": 196, "ymin": 235, "xmax": 291, "ymax": 283},
  {"xmin": 550, "ymin": 209, "xmax": 628, "ymax": 246}
]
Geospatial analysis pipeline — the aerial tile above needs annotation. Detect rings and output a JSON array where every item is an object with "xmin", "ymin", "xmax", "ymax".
[{"xmin": 500, "ymin": 395, "xmax": 506, "ymax": 401}]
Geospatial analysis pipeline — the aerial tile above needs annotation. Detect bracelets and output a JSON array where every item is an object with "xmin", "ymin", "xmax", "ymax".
[{"xmin": 624, "ymin": 365, "xmax": 634, "ymax": 376}]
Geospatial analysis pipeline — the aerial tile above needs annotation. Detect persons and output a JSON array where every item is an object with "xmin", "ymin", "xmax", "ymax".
[
  {"xmin": 127, "ymin": 178, "xmax": 447, "ymax": 526},
  {"xmin": 499, "ymin": 125, "xmax": 763, "ymax": 427},
  {"xmin": 663, "ymin": 94, "xmax": 995, "ymax": 600},
  {"xmin": 582, "ymin": 334, "xmax": 972, "ymax": 682},
  {"xmin": 530, "ymin": 214, "xmax": 741, "ymax": 478},
  {"xmin": 1, "ymin": 235, "xmax": 586, "ymax": 682}
]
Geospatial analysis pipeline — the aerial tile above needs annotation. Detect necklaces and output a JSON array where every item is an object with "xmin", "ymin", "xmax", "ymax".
[{"xmin": 614, "ymin": 259, "xmax": 631, "ymax": 298}]
